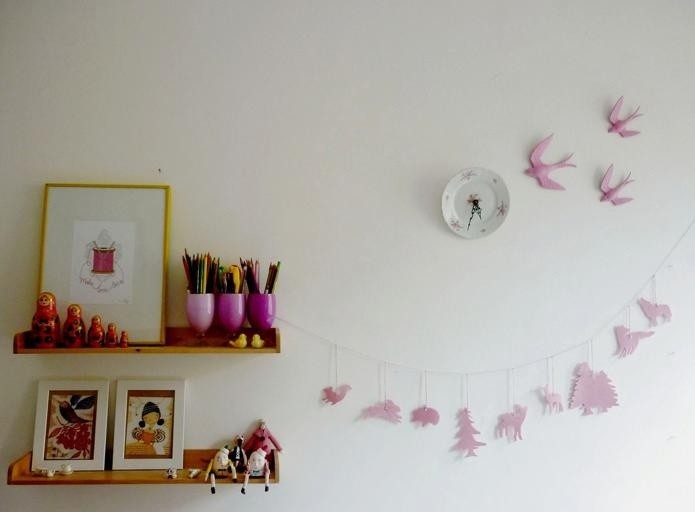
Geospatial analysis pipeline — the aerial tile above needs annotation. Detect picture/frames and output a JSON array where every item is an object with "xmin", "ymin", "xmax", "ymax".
[
  {"xmin": 109, "ymin": 374, "xmax": 189, "ymax": 474},
  {"xmin": 30, "ymin": 377, "xmax": 112, "ymax": 474},
  {"xmin": 37, "ymin": 180, "xmax": 173, "ymax": 347}
]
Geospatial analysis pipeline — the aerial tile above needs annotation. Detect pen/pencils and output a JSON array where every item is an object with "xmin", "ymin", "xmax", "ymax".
[{"xmin": 182, "ymin": 248, "xmax": 280, "ymax": 294}]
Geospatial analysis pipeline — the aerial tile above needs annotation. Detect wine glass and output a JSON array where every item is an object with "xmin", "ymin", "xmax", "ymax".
[
  {"xmin": 185, "ymin": 293, "xmax": 214, "ymax": 347},
  {"xmin": 248, "ymin": 293, "xmax": 276, "ymax": 347},
  {"xmin": 216, "ymin": 293, "xmax": 247, "ymax": 348}
]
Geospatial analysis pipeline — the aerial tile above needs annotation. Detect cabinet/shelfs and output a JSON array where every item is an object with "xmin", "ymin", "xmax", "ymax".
[{"xmin": 5, "ymin": 325, "xmax": 283, "ymax": 489}]
